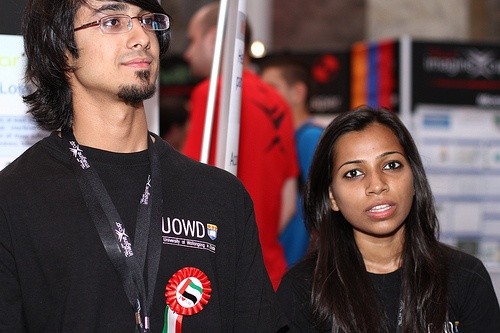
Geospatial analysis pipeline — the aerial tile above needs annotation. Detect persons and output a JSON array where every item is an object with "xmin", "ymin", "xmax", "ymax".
[
  {"xmin": 258, "ymin": 63, "xmax": 329, "ymax": 270},
  {"xmin": 276, "ymin": 105, "xmax": 500, "ymax": 333},
  {"xmin": 0, "ymin": 0, "xmax": 276, "ymax": 333},
  {"xmin": 182, "ymin": 3, "xmax": 300, "ymax": 291}
]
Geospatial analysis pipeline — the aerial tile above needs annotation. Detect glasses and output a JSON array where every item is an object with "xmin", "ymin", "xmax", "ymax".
[{"xmin": 73, "ymin": 12, "xmax": 170, "ymax": 34}]
